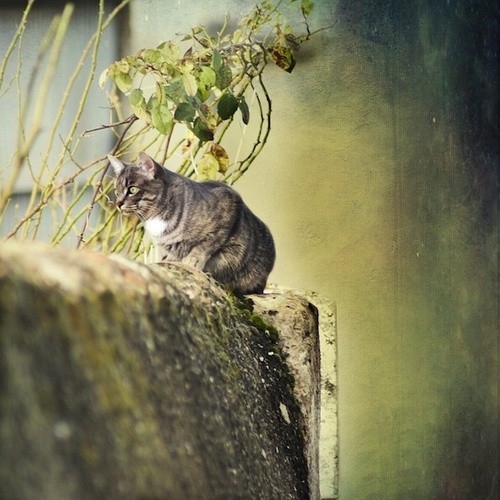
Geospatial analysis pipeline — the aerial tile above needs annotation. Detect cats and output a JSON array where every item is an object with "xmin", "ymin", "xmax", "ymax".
[{"xmin": 106, "ymin": 150, "xmax": 276, "ymax": 300}]
